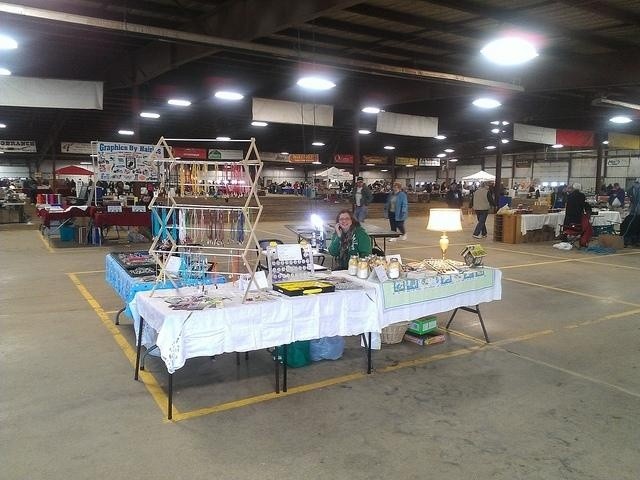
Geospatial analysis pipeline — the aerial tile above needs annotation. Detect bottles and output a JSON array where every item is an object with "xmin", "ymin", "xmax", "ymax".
[
  {"xmin": 318, "ymin": 236, "xmax": 323, "ymax": 253},
  {"xmin": 348, "ymin": 253, "xmax": 399, "ymax": 280},
  {"xmin": 311, "ymin": 232, "xmax": 316, "ymax": 248}
]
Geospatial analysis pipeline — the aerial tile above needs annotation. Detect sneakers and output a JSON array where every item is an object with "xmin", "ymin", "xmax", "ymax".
[
  {"xmin": 481, "ymin": 232, "xmax": 488, "ymax": 238},
  {"xmin": 401, "ymin": 233, "xmax": 407, "ymax": 241},
  {"xmin": 388, "ymin": 237, "xmax": 400, "ymax": 242},
  {"xmin": 472, "ymin": 233, "xmax": 483, "ymax": 239}
]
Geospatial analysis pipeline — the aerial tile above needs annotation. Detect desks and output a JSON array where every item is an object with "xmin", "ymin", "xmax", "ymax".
[
  {"xmin": 283, "ymin": 220, "xmax": 404, "ymax": 265},
  {"xmin": 491, "ymin": 206, "xmax": 623, "ymax": 244},
  {"xmin": 35, "ymin": 204, "xmax": 188, "ymax": 247}
]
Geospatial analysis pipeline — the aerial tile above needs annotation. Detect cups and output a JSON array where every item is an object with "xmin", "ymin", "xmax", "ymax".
[{"xmin": 37, "ymin": 193, "xmax": 76, "ymax": 206}]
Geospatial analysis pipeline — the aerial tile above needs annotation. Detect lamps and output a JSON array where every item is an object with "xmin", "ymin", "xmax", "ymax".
[{"xmin": 426, "ymin": 208, "xmax": 463, "ymax": 261}]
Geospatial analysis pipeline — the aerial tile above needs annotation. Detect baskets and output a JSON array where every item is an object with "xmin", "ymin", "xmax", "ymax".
[{"xmin": 381, "ymin": 321, "xmax": 408, "ymax": 345}]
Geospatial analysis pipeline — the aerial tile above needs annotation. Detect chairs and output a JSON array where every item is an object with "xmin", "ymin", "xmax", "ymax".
[
  {"xmin": 256, "ymin": 239, "xmax": 284, "ymax": 273},
  {"xmin": 558, "ymin": 214, "xmax": 591, "ymax": 250}
]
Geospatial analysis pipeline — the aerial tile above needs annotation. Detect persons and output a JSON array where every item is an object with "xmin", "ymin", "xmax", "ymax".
[
  {"xmin": 619, "ymin": 182, "xmax": 640, "ymax": 247},
  {"xmin": 350, "ymin": 177, "xmax": 373, "ymax": 223},
  {"xmin": 469, "ymin": 181, "xmax": 494, "ymax": 239},
  {"xmin": 382, "ymin": 182, "xmax": 408, "ymax": 242},
  {"xmin": 337, "ymin": 181, "xmax": 350, "ymax": 199},
  {"xmin": 421, "ymin": 180, "xmax": 480, "ymax": 199},
  {"xmin": 369, "ymin": 180, "xmax": 392, "ymax": 193},
  {"xmin": 551, "ymin": 185, "xmax": 568, "ymax": 208},
  {"xmin": 328, "ymin": 208, "xmax": 372, "ymax": 271},
  {"xmin": 596, "ymin": 183, "xmax": 625, "ymax": 208},
  {"xmin": 489, "ymin": 183, "xmax": 540, "ymax": 200},
  {"xmin": 0, "ymin": 177, "xmax": 134, "ymax": 201},
  {"xmin": 561, "ymin": 183, "xmax": 592, "ymax": 250},
  {"xmin": 268, "ymin": 180, "xmax": 308, "ymax": 196}
]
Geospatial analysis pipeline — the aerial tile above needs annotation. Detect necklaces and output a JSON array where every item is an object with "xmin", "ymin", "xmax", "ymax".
[{"xmin": 184, "ymin": 163, "xmax": 247, "ymax": 246}]
